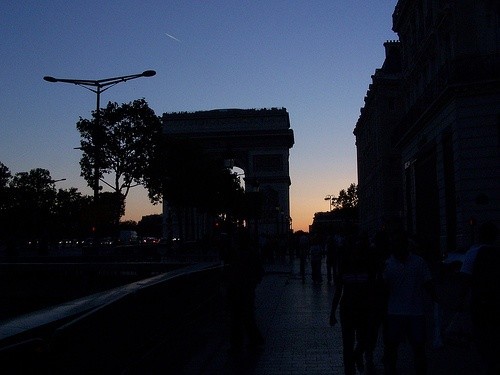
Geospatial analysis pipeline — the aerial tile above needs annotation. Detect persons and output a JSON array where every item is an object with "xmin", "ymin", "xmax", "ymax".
[{"xmin": 252, "ymin": 220, "xmax": 500, "ymax": 375}]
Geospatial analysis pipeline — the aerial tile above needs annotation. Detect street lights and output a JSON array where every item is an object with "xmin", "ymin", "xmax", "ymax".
[
  {"xmin": 45, "ymin": 70, "xmax": 158, "ymax": 262},
  {"xmin": 325, "ymin": 195, "xmax": 336, "ymax": 212}
]
